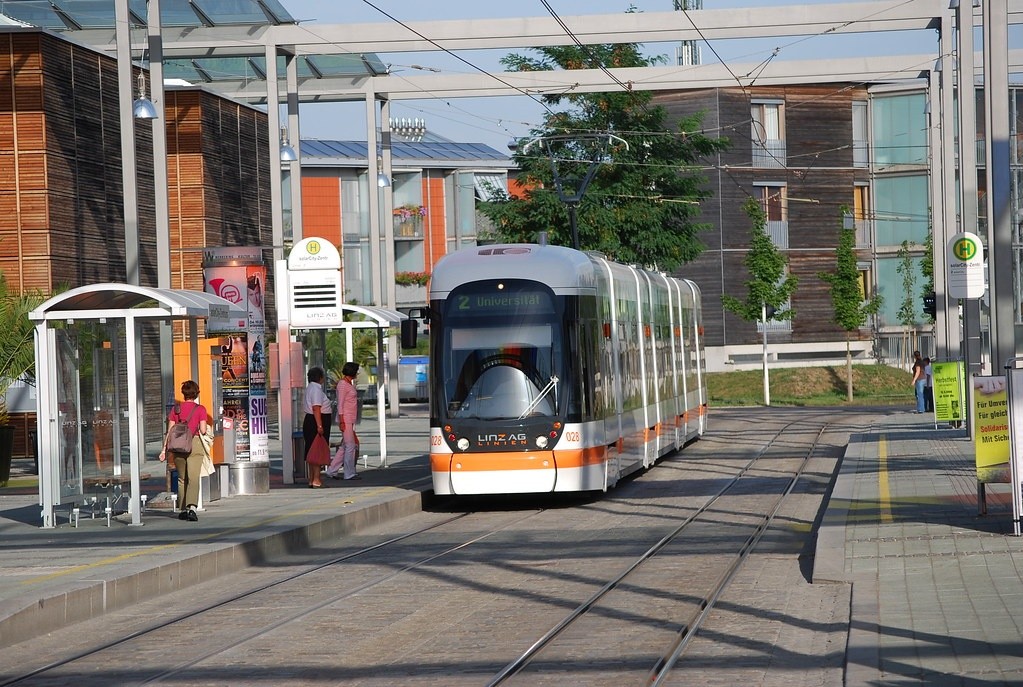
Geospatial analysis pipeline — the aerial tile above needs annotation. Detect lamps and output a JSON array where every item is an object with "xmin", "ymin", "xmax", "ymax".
[
  {"xmin": 948, "ymin": 0, "xmax": 980, "ymax": 9},
  {"xmin": 935, "ymin": 57, "xmax": 956, "ymax": 72},
  {"xmin": 388, "ymin": 118, "xmax": 426, "ymax": 142},
  {"xmin": 377, "ymin": 155, "xmax": 391, "ymax": 187},
  {"xmin": 279, "ymin": 122, "xmax": 297, "ymax": 161},
  {"xmin": 130, "ymin": 26, "xmax": 158, "ymax": 119}
]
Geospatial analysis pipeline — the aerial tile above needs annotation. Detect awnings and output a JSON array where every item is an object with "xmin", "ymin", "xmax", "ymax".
[{"xmin": 474, "ymin": 175, "xmax": 511, "ymax": 202}]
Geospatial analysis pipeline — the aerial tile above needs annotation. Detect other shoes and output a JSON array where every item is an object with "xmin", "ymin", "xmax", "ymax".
[
  {"xmin": 914, "ymin": 411, "xmax": 924, "ymax": 414},
  {"xmin": 350, "ymin": 475, "xmax": 362, "ymax": 480},
  {"xmin": 325, "ymin": 473, "xmax": 344, "ymax": 481}
]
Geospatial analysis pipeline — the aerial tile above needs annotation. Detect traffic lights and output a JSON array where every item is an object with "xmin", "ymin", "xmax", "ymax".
[{"xmin": 923, "ymin": 293, "xmax": 936, "ymax": 319}]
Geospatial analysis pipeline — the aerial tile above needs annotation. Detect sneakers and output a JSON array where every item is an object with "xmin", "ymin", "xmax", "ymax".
[{"xmin": 179, "ymin": 510, "xmax": 198, "ymax": 521}]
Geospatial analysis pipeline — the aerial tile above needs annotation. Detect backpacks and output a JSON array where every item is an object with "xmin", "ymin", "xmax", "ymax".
[{"xmin": 166, "ymin": 403, "xmax": 200, "ymax": 453}]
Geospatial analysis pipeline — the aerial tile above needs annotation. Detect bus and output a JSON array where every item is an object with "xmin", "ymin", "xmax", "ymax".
[{"xmin": 401, "ymin": 235, "xmax": 708, "ymax": 504}]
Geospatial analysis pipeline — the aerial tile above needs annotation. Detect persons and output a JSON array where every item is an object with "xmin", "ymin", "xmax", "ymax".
[
  {"xmin": 252, "ymin": 335, "xmax": 262, "ymax": 372},
  {"xmin": 248, "ymin": 276, "xmax": 264, "ymax": 319},
  {"xmin": 158, "ymin": 380, "xmax": 208, "ymax": 522},
  {"xmin": 923, "ymin": 358, "xmax": 934, "ymax": 412},
  {"xmin": 952, "ymin": 401, "xmax": 959, "ymax": 413},
  {"xmin": 63, "ymin": 404, "xmax": 77, "ymax": 483},
  {"xmin": 911, "ymin": 351, "xmax": 926, "ymax": 414},
  {"xmin": 302, "ymin": 367, "xmax": 333, "ymax": 489},
  {"xmin": 326, "ymin": 363, "xmax": 362, "ymax": 480}
]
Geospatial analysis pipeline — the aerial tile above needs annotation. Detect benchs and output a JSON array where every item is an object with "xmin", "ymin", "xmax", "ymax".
[
  {"xmin": 82, "ymin": 473, "xmax": 151, "ymax": 521},
  {"xmin": 322, "ymin": 443, "xmax": 341, "ymax": 471}
]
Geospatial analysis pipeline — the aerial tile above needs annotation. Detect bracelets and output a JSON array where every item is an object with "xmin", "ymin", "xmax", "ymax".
[
  {"xmin": 318, "ymin": 426, "xmax": 322, "ymax": 428},
  {"xmin": 340, "ymin": 422, "xmax": 345, "ymax": 424},
  {"xmin": 161, "ymin": 451, "xmax": 165, "ymax": 453}
]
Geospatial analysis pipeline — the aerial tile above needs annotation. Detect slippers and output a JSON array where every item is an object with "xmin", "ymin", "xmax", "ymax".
[{"xmin": 308, "ymin": 483, "xmax": 329, "ymax": 489}]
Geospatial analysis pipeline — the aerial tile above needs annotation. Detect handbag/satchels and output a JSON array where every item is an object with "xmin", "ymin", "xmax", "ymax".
[
  {"xmin": 306, "ymin": 432, "xmax": 330, "ymax": 466},
  {"xmin": 336, "ymin": 437, "xmax": 359, "ymax": 467}
]
